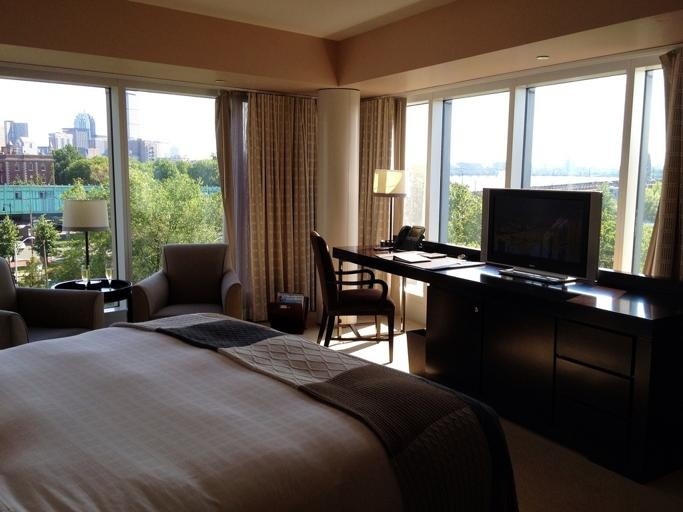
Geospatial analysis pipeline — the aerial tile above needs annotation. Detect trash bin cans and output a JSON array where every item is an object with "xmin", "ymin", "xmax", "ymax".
[{"xmin": 406, "ymin": 328, "xmax": 441, "ymax": 377}]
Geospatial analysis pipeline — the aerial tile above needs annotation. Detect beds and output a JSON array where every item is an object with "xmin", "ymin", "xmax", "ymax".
[{"xmin": 0, "ymin": 310, "xmax": 505, "ymax": 512}]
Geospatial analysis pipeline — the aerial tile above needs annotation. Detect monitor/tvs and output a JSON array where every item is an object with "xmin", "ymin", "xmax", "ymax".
[{"xmin": 480, "ymin": 186, "xmax": 603, "ymax": 285}]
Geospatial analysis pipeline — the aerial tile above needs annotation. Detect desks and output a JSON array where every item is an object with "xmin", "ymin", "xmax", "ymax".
[{"xmin": 332, "ymin": 241, "xmax": 682, "ymax": 486}]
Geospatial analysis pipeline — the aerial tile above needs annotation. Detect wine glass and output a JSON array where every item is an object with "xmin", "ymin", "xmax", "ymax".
[
  {"xmin": 80, "ymin": 264, "xmax": 91, "ymax": 290},
  {"xmin": 105, "ymin": 264, "xmax": 117, "ymax": 289}
]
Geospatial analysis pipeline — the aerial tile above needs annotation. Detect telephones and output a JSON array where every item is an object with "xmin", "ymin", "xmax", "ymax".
[{"xmin": 393, "ymin": 225, "xmax": 426, "ymax": 251}]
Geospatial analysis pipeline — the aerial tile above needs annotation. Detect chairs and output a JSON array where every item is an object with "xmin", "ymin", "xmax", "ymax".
[
  {"xmin": 308, "ymin": 230, "xmax": 395, "ymax": 364},
  {"xmin": 0, "ymin": 243, "xmax": 242, "ymax": 349}
]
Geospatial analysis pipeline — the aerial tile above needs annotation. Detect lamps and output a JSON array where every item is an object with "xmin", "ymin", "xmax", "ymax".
[
  {"xmin": 371, "ymin": 168, "xmax": 407, "ymax": 241},
  {"xmin": 62, "ymin": 198, "xmax": 110, "ymax": 267}
]
